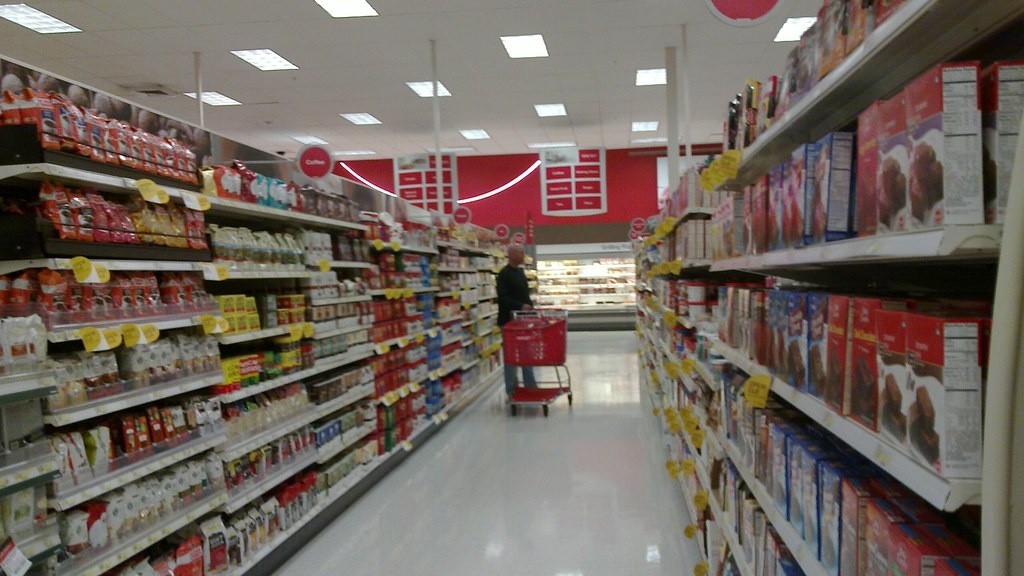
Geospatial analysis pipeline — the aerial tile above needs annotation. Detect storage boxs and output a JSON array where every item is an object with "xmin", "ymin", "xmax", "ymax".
[
  {"xmin": 0, "ymin": 231, "xmax": 514, "ymax": 576},
  {"xmin": 637, "ymin": 0, "xmax": 1024, "ymax": 576}
]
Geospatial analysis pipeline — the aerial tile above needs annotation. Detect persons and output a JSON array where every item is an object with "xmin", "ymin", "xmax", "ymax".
[{"xmin": 496, "ymin": 243, "xmax": 538, "ymax": 400}]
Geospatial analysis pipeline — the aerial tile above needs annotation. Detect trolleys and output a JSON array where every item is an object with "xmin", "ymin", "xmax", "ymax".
[{"xmin": 502, "ymin": 309, "xmax": 573, "ymax": 417}]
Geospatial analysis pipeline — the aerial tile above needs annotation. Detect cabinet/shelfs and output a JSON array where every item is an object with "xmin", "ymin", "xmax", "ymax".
[
  {"xmin": 0, "ymin": 121, "xmax": 237, "ymax": 576},
  {"xmin": 361, "ymin": 225, "xmax": 445, "ymax": 486},
  {"xmin": 204, "ymin": 191, "xmax": 373, "ymax": 576},
  {"xmin": 702, "ymin": 0, "xmax": 1024, "ymax": 576},
  {"xmin": 438, "ymin": 241, "xmax": 535, "ymax": 418},
  {"xmin": 639, "ymin": 207, "xmax": 712, "ymax": 541}
]
